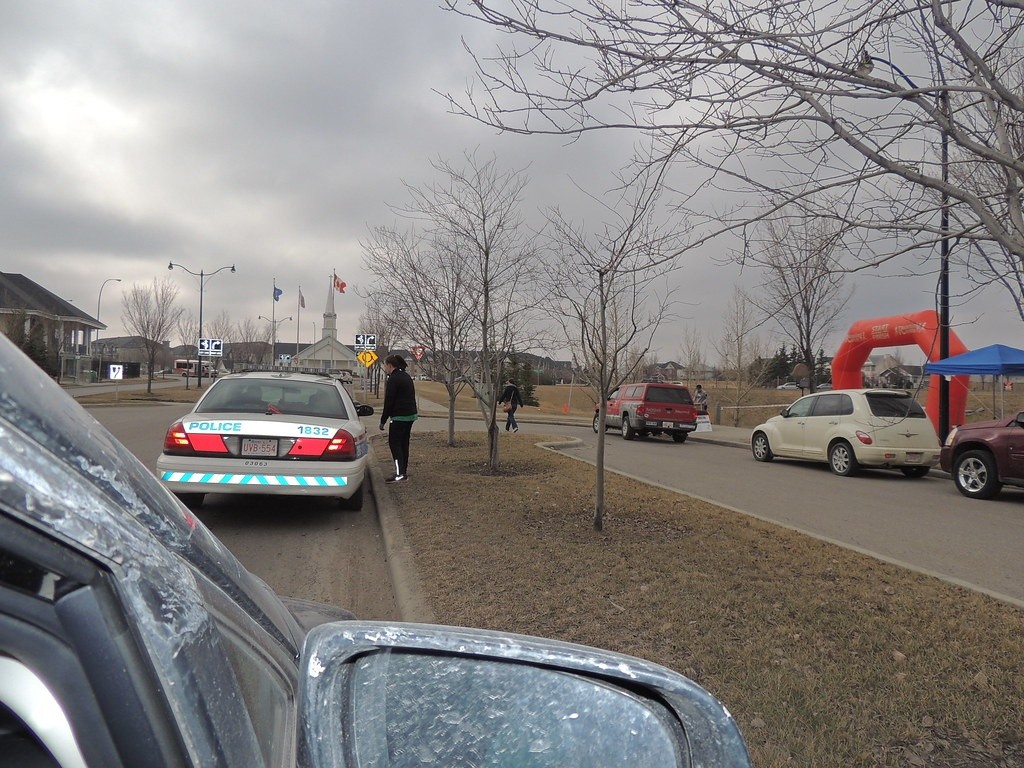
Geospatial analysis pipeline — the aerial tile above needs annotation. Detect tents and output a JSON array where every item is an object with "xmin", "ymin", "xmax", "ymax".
[{"xmin": 922, "ymin": 343, "xmax": 1024, "ymax": 420}]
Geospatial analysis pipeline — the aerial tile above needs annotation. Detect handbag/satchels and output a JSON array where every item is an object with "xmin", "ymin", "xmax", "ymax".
[{"xmin": 502, "ymin": 402, "xmax": 512, "ymax": 412}]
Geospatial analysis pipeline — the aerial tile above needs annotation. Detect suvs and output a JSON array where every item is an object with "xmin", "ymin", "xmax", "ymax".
[{"xmin": 592, "ymin": 383, "xmax": 698, "ymax": 443}]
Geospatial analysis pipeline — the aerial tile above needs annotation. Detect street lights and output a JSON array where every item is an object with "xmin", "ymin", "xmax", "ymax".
[
  {"xmin": 167, "ymin": 262, "xmax": 236, "ymax": 387},
  {"xmin": 858, "ymin": 51, "xmax": 955, "ymax": 448},
  {"xmin": 95, "ymin": 278, "xmax": 122, "ymax": 356},
  {"xmin": 258, "ymin": 315, "xmax": 293, "ymax": 370}
]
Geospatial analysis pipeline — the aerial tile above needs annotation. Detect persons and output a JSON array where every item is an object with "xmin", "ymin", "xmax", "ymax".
[
  {"xmin": 692, "ymin": 385, "xmax": 708, "ymax": 413},
  {"xmin": 498, "ymin": 378, "xmax": 524, "ymax": 433},
  {"xmin": 379, "ymin": 354, "xmax": 418, "ymax": 483}
]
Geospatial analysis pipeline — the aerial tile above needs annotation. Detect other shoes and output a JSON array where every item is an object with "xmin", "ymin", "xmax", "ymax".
[
  {"xmin": 384, "ymin": 473, "xmax": 409, "ymax": 483},
  {"xmin": 504, "ymin": 428, "xmax": 510, "ymax": 431},
  {"xmin": 512, "ymin": 428, "xmax": 519, "ymax": 433}
]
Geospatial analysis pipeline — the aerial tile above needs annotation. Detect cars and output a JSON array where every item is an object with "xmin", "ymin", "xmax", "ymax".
[
  {"xmin": 156, "ymin": 369, "xmax": 374, "ymax": 512},
  {"xmin": 0, "ymin": 334, "xmax": 760, "ymax": 767},
  {"xmin": 940, "ymin": 410, "xmax": 1024, "ymax": 500},
  {"xmin": 330, "ymin": 369, "xmax": 354, "ymax": 384},
  {"xmin": 817, "ymin": 383, "xmax": 833, "ymax": 389},
  {"xmin": 749, "ymin": 388, "xmax": 943, "ymax": 479},
  {"xmin": 776, "ymin": 381, "xmax": 799, "ymax": 390}
]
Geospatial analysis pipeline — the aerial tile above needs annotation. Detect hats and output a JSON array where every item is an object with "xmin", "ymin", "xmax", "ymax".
[{"xmin": 695, "ymin": 385, "xmax": 701, "ymax": 389}]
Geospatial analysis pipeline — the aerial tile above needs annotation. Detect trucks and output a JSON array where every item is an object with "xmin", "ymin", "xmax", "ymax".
[{"xmin": 173, "ymin": 359, "xmax": 218, "ymax": 378}]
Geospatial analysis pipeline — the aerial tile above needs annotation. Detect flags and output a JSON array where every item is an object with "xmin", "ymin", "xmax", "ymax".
[
  {"xmin": 334, "ymin": 274, "xmax": 346, "ymax": 293},
  {"xmin": 273, "ymin": 285, "xmax": 282, "ymax": 301},
  {"xmin": 299, "ymin": 291, "xmax": 306, "ymax": 308}
]
